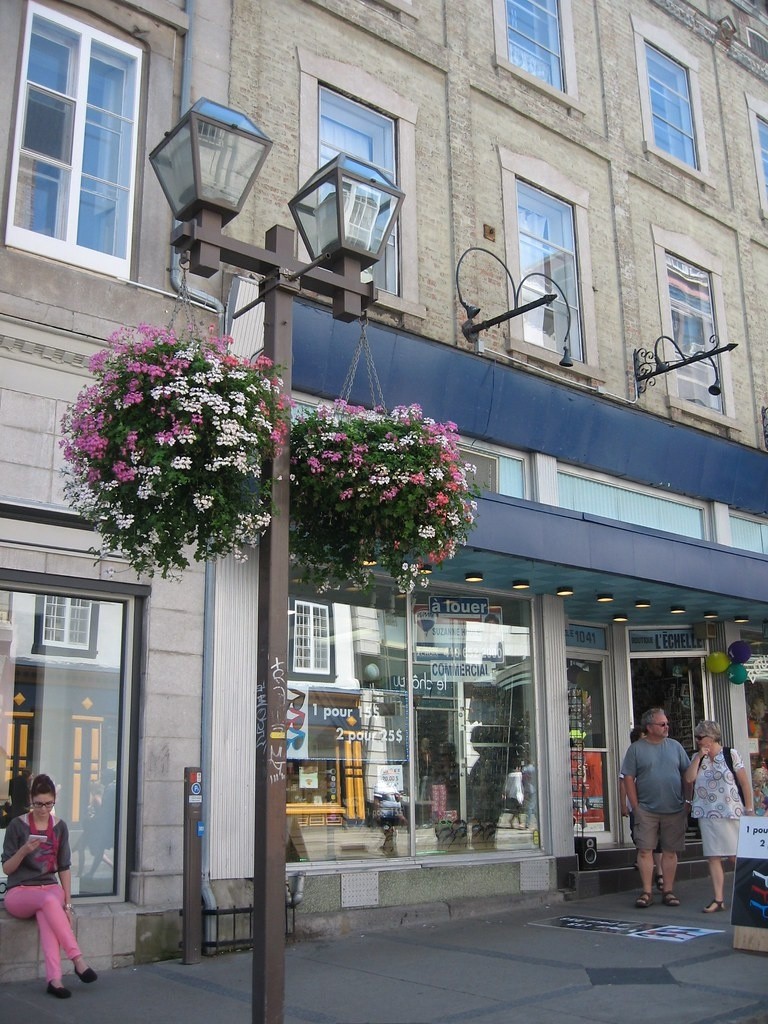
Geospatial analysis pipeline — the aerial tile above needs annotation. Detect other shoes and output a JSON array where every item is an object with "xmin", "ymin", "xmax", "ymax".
[
  {"xmin": 74, "ymin": 965, "xmax": 98, "ymax": 983},
  {"xmin": 47, "ymin": 984, "xmax": 72, "ymax": 999}
]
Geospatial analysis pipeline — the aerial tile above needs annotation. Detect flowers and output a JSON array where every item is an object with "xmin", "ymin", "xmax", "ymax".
[
  {"xmin": 287, "ymin": 396, "xmax": 480, "ymax": 595},
  {"xmin": 56, "ymin": 322, "xmax": 295, "ymax": 586}
]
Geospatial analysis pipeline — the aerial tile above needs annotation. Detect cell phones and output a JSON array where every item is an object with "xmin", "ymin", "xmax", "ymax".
[{"xmin": 28, "ymin": 834, "xmax": 48, "ymax": 843}]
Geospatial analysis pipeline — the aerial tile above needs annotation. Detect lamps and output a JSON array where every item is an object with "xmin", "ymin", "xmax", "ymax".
[
  {"xmin": 457, "ymin": 247, "xmax": 574, "ymax": 368},
  {"xmin": 597, "ymin": 594, "xmax": 613, "ymax": 601},
  {"xmin": 704, "ymin": 611, "xmax": 719, "ymax": 618},
  {"xmin": 635, "ymin": 600, "xmax": 651, "ymax": 608},
  {"xmin": 613, "ymin": 614, "xmax": 628, "ymax": 621},
  {"xmin": 632, "ymin": 335, "xmax": 738, "ymax": 399},
  {"xmin": 419, "ymin": 564, "xmax": 433, "ymax": 575},
  {"xmin": 557, "ymin": 587, "xmax": 573, "ymax": 595},
  {"xmin": 671, "ymin": 606, "xmax": 686, "ymax": 613},
  {"xmin": 733, "ymin": 615, "xmax": 749, "ymax": 623},
  {"xmin": 512, "ymin": 580, "xmax": 530, "ymax": 589},
  {"xmin": 465, "ymin": 573, "xmax": 483, "ymax": 582}
]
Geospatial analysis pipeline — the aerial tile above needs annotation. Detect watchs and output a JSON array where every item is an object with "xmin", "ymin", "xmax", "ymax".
[{"xmin": 685, "ymin": 799, "xmax": 693, "ymax": 805}]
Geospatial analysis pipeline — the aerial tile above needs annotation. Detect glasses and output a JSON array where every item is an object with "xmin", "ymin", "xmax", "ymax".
[
  {"xmin": 286, "ymin": 708, "xmax": 305, "ymax": 731},
  {"xmin": 652, "ymin": 722, "xmax": 670, "ymax": 727},
  {"xmin": 32, "ymin": 799, "xmax": 56, "ymax": 807},
  {"xmin": 286, "ymin": 689, "xmax": 306, "ymax": 711},
  {"xmin": 286, "ymin": 727, "xmax": 305, "ymax": 750},
  {"xmin": 694, "ymin": 735, "xmax": 708, "ymax": 741},
  {"xmin": 750, "ymin": 870, "xmax": 768, "ymax": 919}
]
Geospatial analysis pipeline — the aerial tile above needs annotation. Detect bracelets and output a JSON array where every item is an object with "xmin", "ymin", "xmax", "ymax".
[
  {"xmin": 64, "ymin": 903, "xmax": 72, "ymax": 911},
  {"xmin": 695, "ymin": 754, "xmax": 700, "ymax": 763},
  {"xmin": 745, "ymin": 808, "xmax": 754, "ymax": 812}
]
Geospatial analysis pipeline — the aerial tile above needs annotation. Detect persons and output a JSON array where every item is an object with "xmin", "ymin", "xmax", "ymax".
[
  {"xmin": 1, "ymin": 774, "xmax": 97, "ymax": 999},
  {"xmin": 371, "ymin": 771, "xmax": 410, "ymax": 856},
  {"xmin": 434, "ymin": 724, "xmax": 503, "ymax": 852},
  {"xmin": 418, "ymin": 738, "xmax": 450, "ymax": 829},
  {"xmin": 619, "ymin": 726, "xmax": 664, "ymax": 891},
  {"xmin": 684, "ymin": 720, "xmax": 758, "ymax": 913},
  {"xmin": 621, "ymin": 708, "xmax": 692, "ymax": 907},
  {"xmin": 502, "ymin": 758, "xmax": 540, "ymax": 830},
  {"xmin": 9, "ymin": 769, "xmax": 32, "ymax": 820}
]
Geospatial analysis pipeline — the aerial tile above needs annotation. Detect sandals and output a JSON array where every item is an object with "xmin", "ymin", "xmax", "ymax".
[
  {"xmin": 636, "ymin": 891, "xmax": 654, "ymax": 906},
  {"xmin": 662, "ymin": 891, "xmax": 680, "ymax": 906},
  {"xmin": 634, "ymin": 862, "xmax": 638, "ymax": 869},
  {"xmin": 703, "ymin": 899, "xmax": 725, "ymax": 914},
  {"xmin": 656, "ymin": 872, "xmax": 664, "ymax": 891}
]
